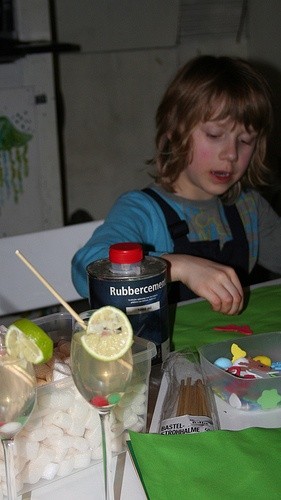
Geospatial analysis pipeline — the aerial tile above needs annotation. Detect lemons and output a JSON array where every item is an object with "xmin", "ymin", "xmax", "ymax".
[
  {"xmin": 81, "ymin": 306, "xmax": 134, "ymax": 362},
  {"xmin": 3, "ymin": 317, "xmax": 54, "ymax": 364}
]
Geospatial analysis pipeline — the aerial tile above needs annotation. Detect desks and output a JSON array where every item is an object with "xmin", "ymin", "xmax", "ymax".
[{"xmin": 0, "ymin": 277, "xmax": 281, "ymax": 500}]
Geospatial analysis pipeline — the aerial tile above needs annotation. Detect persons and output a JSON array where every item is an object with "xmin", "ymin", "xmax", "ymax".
[{"xmin": 71, "ymin": 51, "xmax": 279, "ymax": 316}]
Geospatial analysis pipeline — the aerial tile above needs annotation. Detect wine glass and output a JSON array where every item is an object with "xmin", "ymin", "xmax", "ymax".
[
  {"xmin": 68, "ymin": 310, "xmax": 135, "ymax": 463},
  {"xmin": 0, "ymin": 346, "xmax": 38, "ymax": 500}
]
